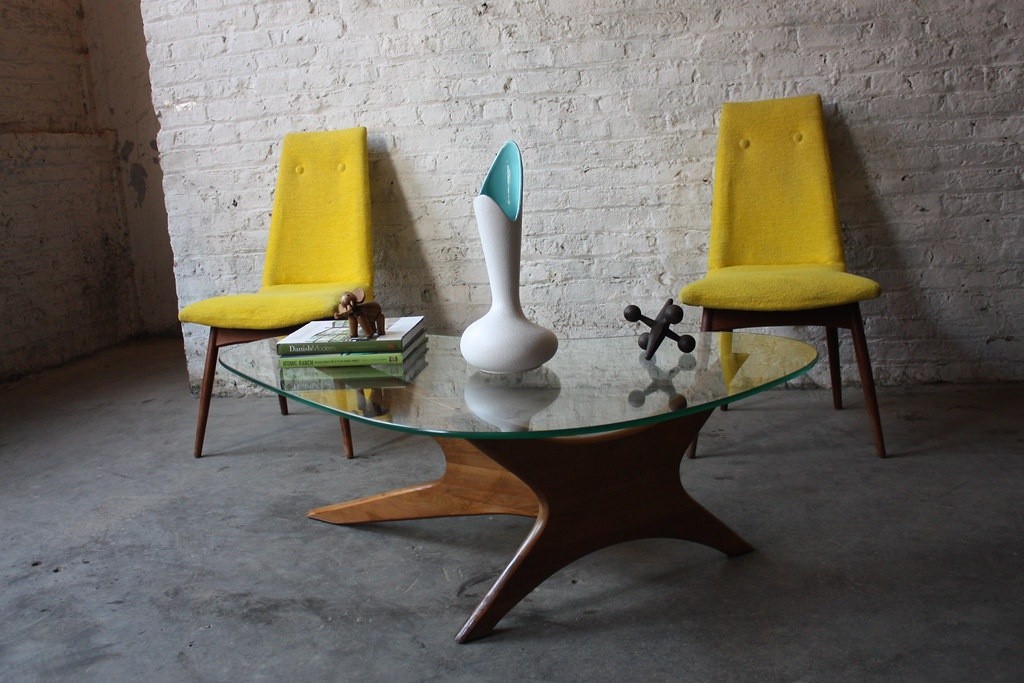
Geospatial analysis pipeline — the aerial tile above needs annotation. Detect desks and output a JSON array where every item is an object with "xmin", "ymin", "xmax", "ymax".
[{"xmin": 220, "ymin": 331, "xmax": 820, "ymax": 647}]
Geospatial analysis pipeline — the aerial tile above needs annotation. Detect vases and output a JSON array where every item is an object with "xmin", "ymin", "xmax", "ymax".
[{"xmin": 458, "ymin": 142, "xmax": 558, "ymax": 375}]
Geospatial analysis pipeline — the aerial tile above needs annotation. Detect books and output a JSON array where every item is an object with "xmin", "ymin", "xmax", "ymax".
[
  {"xmin": 280, "ymin": 343, "xmax": 430, "ymax": 391},
  {"xmin": 276, "ymin": 316, "xmax": 428, "ymax": 367}
]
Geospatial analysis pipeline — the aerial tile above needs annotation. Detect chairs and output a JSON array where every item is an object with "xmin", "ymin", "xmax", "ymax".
[
  {"xmin": 179, "ymin": 128, "xmax": 374, "ymax": 460},
  {"xmin": 680, "ymin": 94, "xmax": 887, "ymax": 461}
]
